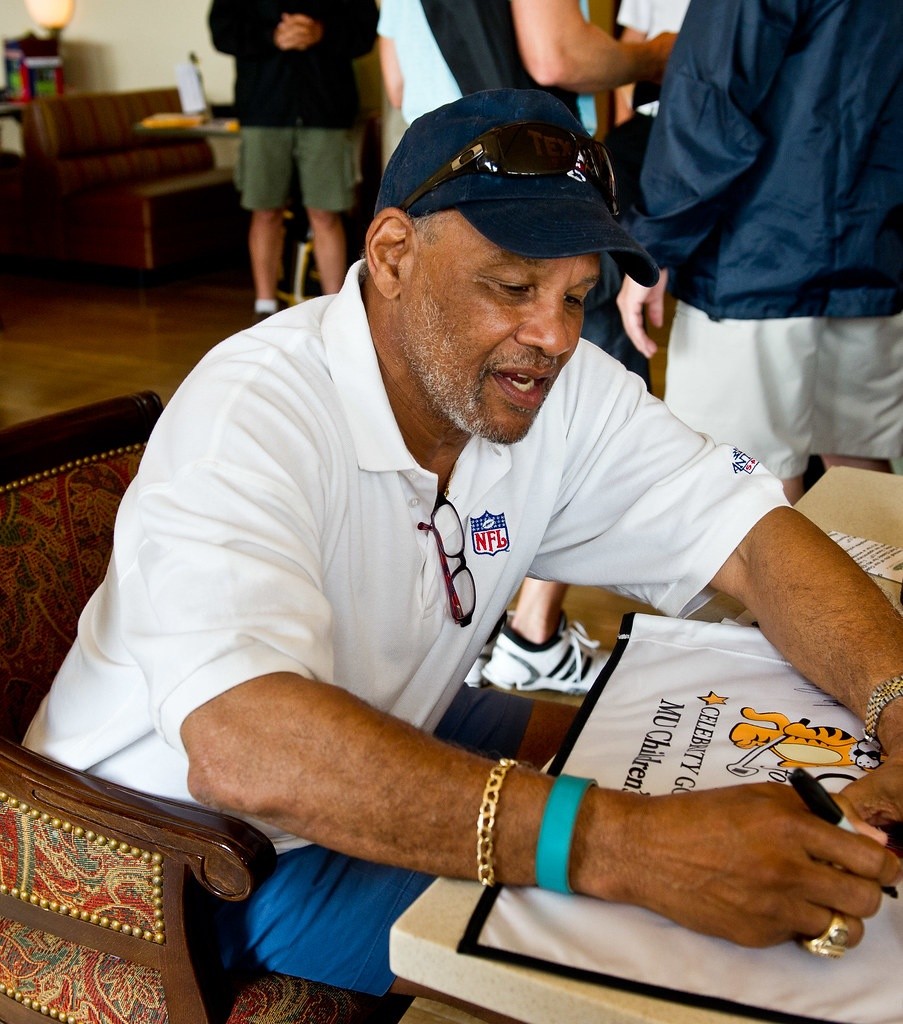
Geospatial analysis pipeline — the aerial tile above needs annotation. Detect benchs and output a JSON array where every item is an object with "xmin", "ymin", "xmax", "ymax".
[{"xmin": 21, "ymin": 85, "xmax": 215, "ymax": 274}]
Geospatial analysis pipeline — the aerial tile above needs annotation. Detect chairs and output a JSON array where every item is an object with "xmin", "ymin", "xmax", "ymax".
[{"xmin": 0, "ymin": 384, "xmax": 430, "ymax": 1024}]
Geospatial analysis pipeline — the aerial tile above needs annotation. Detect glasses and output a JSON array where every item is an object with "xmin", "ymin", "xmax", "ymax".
[
  {"xmin": 418, "ymin": 493, "xmax": 476, "ymax": 628},
  {"xmin": 396, "ymin": 121, "xmax": 622, "ymax": 215}
]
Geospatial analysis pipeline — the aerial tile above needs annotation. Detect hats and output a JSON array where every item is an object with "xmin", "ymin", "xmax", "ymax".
[{"xmin": 373, "ymin": 89, "xmax": 661, "ymax": 288}]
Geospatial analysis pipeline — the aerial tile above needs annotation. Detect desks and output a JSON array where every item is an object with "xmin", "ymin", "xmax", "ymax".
[
  {"xmin": 389, "ymin": 466, "xmax": 903, "ymax": 1024},
  {"xmin": 137, "ymin": 110, "xmax": 242, "ymax": 145}
]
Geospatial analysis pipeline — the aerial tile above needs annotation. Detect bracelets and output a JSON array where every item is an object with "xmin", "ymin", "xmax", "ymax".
[
  {"xmin": 533, "ymin": 774, "xmax": 598, "ymax": 895},
  {"xmin": 863, "ymin": 675, "xmax": 903, "ymax": 750},
  {"xmin": 477, "ymin": 758, "xmax": 519, "ymax": 886}
]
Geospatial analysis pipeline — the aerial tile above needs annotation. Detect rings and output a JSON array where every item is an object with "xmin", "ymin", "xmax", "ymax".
[{"xmin": 801, "ymin": 910, "xmax": 849, "ymax": 959}]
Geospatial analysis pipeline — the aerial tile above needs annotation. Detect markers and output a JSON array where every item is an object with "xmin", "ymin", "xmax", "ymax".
[{"xmin": 787, "ymin": 769, "xmax": 899, "ymax": 898}]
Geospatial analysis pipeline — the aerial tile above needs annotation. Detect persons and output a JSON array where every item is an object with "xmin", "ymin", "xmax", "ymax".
[
  {"xmin": 24, "ymin": 89, "xmax": 903, "ymax": 1024},
  {"xmin": 206, "ymin": 0, "xmax": 379, "ymax": 336},
  {"xmin": 378, "ymin": 0, "xmax": 903, "ymax": 695}
]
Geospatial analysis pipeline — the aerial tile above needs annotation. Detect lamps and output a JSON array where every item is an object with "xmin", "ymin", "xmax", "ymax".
[{"xmin": 25, "ymin": 1, "xmax": 76, "ymax": 40}]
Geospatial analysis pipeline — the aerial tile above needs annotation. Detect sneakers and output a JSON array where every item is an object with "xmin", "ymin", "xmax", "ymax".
[{"xmin": 481, "ymin": 609, "xmax": 612, "ymax": 695}]
